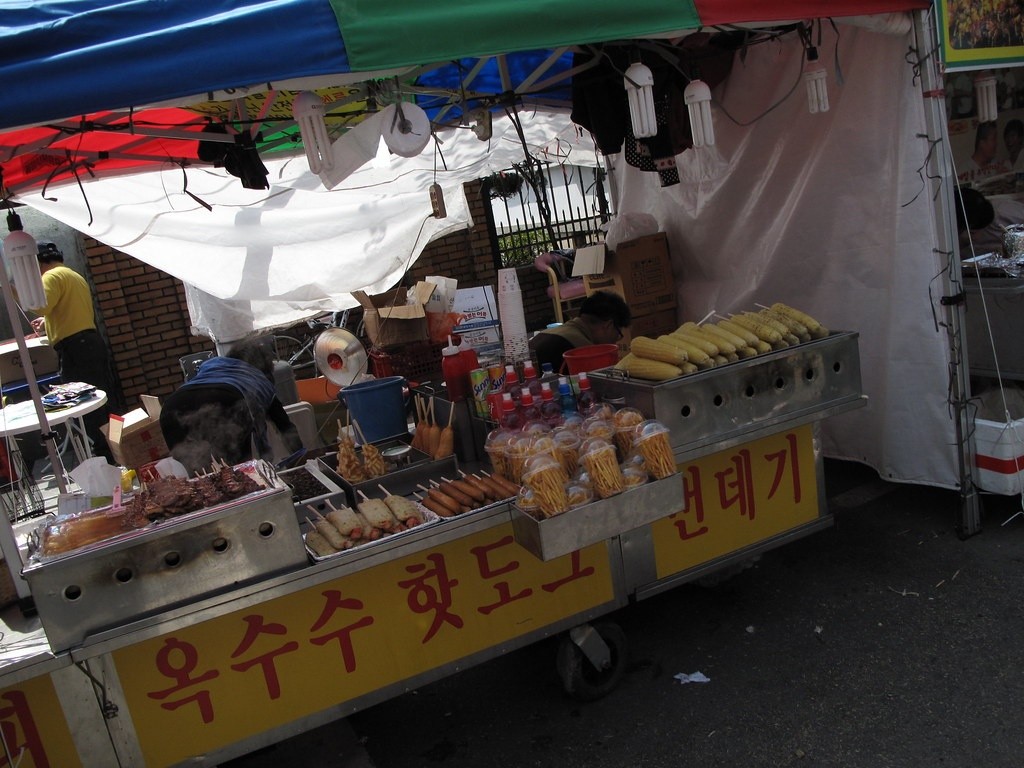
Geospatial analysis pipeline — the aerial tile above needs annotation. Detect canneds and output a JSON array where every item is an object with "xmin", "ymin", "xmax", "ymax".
[{"xmin": 471, "ymin": 364, "xmax": 506, "ymax": 418}]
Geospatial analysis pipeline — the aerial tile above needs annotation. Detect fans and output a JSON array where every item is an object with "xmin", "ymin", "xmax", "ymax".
[{"xmin": 313, "ymin": 326, "xmax": 368, "ymax": 387}]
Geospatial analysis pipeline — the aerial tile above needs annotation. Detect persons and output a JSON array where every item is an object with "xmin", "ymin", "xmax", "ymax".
[
  {"xmin": 159, "ymin": 343, "xmax": 303, "ymax": 479},
  {"xmin": 30, "ymin": 243, "xmax": 120, "ymax": 465},
  {"xmin": 948, "ymin": 68, "xmax": 1015, "ymax": 117},
  {"xmin": 955, "ymin": 188, "xmax": 1024, "ymax": 262},
  {"xmin": 529, "ymin": 291, "xmax": 631, "ymax": 374},
  {"xmin": 958, "ymin": 121, "xmax": 998, "ymax": 176},
  {"xmin": 1003, "ymin": 119, "xmax": 1024, "ymax": 171}
]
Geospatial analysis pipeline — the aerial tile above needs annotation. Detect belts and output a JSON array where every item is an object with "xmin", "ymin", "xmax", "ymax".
[{"xmin": 55, "ymin": 330, "xmax": 95, "ymax": 350}]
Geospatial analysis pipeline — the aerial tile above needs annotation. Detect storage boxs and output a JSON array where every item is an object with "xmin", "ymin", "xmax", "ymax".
[
  {"xmin": 571, "ymin": 231, "xmax": 677, "ymax": 318},
  {"xmin": 0, "ymin": 336, "xmax": 59, "ymax": 383},
  {"xmin": 349, "ymin": 280, "xmax": 437, "ymax": 349},
  {"xmin": 972, "ymin": 393, "xmax": 1024, "ymax": 496},
  {"xmin": 451, "ymin": 285, "xmax": 499, "ymax": 334},
  {"xmin": 424, "ymin": 275, "xmax": 458, "ymax": 313},
  {"xmin": 366, "ymin": 338, "xmax": 462, "ymax": 384},
  {"xmin": 57, "ymin": 493, "xmax": 113, "ymax": 515},
  {"xmin": 632, "ymin": 308, "xmax": 677, "ymax": 339},
  {"xmin": 98, "ymin": 393, "xmax": 170, "ymax": 468},
  {"xmin": 459, "ymin": 327, "xmax": 502, "ymax": 363}
]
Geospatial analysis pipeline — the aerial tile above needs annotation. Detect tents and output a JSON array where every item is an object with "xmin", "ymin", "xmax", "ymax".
[{"xmin": 0, "ymin": 0, "xmax": 976, "ymax": 539}]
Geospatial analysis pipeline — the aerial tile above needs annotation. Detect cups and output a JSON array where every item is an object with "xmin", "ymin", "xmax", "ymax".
[
  {"xmin": 497, "ymin": 268, "xmax": 529, "ymax": 364},
  {"xmin": 482, "ymin": 403, "xmax": 679, "ymax": 518}
]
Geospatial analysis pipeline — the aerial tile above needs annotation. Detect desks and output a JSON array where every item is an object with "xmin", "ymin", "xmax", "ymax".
[{"xmin": 0, "ymin": 389, "xmax": 108, "ymax": 484}]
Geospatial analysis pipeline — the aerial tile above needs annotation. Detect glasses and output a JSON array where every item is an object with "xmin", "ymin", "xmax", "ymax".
[{"xmin": 602, "ymin": 319, "xmax": 624, "ymax": 341}]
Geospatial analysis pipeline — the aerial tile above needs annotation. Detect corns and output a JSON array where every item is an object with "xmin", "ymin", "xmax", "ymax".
[
  {"xmin": 612, "ymin": 302, "xmax": 830, "ymax": 380},
  {"xmin": 410, "ymin": 421, "xmax": 453, "ymax": 459}
]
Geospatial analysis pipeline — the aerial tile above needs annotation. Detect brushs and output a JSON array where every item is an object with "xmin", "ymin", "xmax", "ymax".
[{"xmin": 106, "ymin": 484, "xmax": 126, "ymax": 519}]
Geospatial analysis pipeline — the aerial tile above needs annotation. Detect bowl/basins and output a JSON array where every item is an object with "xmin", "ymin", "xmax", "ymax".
[{"xmin": 382, "ymin": 445, "xmax": 411, "ymax": 460}]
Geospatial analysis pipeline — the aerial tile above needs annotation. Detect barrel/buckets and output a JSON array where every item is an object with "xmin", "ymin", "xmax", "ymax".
[
  {"xmin": 340, "ymin": 376, "xmax": 408, "ymax": 446},
  {"xmin": 564, "ymin": 344, "xmax": 620, "ymax": 376}
]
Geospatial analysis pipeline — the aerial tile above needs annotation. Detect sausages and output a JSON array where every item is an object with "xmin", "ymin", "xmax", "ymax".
[{"xmin": 421, "ymin": 472, "xmax": 520, "ymax": 516}]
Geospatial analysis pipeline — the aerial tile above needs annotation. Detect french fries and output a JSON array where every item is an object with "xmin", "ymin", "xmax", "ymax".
[{"xmin": 490, "ymin": 405, "xmax": 679, "ymax": 518}]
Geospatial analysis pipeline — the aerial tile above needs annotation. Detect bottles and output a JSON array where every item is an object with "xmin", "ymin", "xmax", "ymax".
[{"xmin": 437, "ymin": 334, "xmax": 597, "ymax": 431}]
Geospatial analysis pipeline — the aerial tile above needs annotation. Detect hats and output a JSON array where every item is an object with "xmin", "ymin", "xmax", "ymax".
[{"xmin": 37, "ymin": 240, "xmax": 57, "ymax": 254}]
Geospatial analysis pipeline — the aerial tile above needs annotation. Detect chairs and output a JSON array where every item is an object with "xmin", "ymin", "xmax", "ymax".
[{"xmin": 534, "ymin": 253, "xmax": 587, "ymax": 324}]
